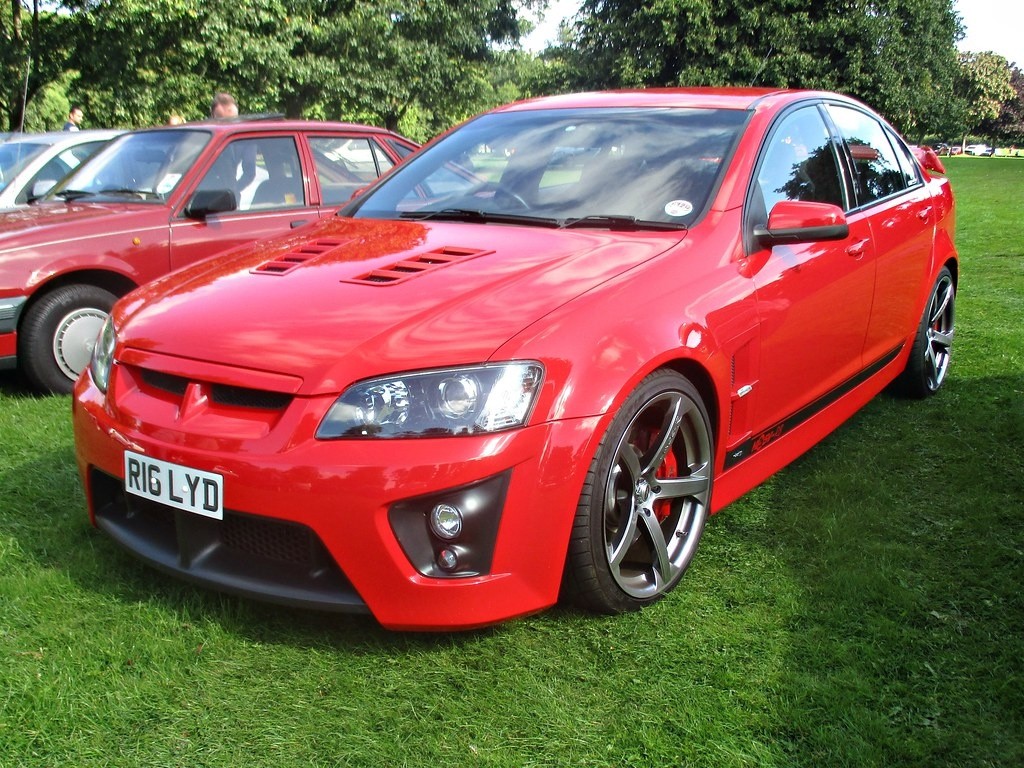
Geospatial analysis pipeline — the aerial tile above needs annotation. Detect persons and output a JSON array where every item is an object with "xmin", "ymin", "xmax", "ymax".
[
  {"xmin": 169, "ymin": 115, "xmax": 186, "ymax": 125},
  {"xmin": 63, "ymin": 106, "xmax": 84, "ymax": 131},
  {"xmin": 197, "ymin": 94, "xmax": 256, "ymax": 208},
  {"xmin": 1015, "ymin": 150, "xmax": 1020, "ymax": 156},
  {"xmin": 1009, "ymin": 146, "xmax": 1013, "ymax": 154}
]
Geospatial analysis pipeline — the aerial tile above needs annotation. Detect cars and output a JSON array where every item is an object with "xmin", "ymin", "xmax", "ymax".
[
  {"xmin": 0, "ymin": 129, "xmax": 270, "ymax": 210},
  {"xmin": 0, "ymin": 113, "xmax": 498, "ymax": 397},
  {"xmin": 932, "ymin": 144, "xmax": 1005, "ymax": 156},
  {"xmin": 73, "ymin": 87, "xmax": 961, "ymax": 634}
]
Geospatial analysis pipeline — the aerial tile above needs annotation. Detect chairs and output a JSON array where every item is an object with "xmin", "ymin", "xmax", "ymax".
[
  {"xmin": 551, "ymin": 153, "xmax": 634, "ymax": 218},
  {"xmin": 753, "ymin": 142, "xmax": 814, "ymax": 228}
]
